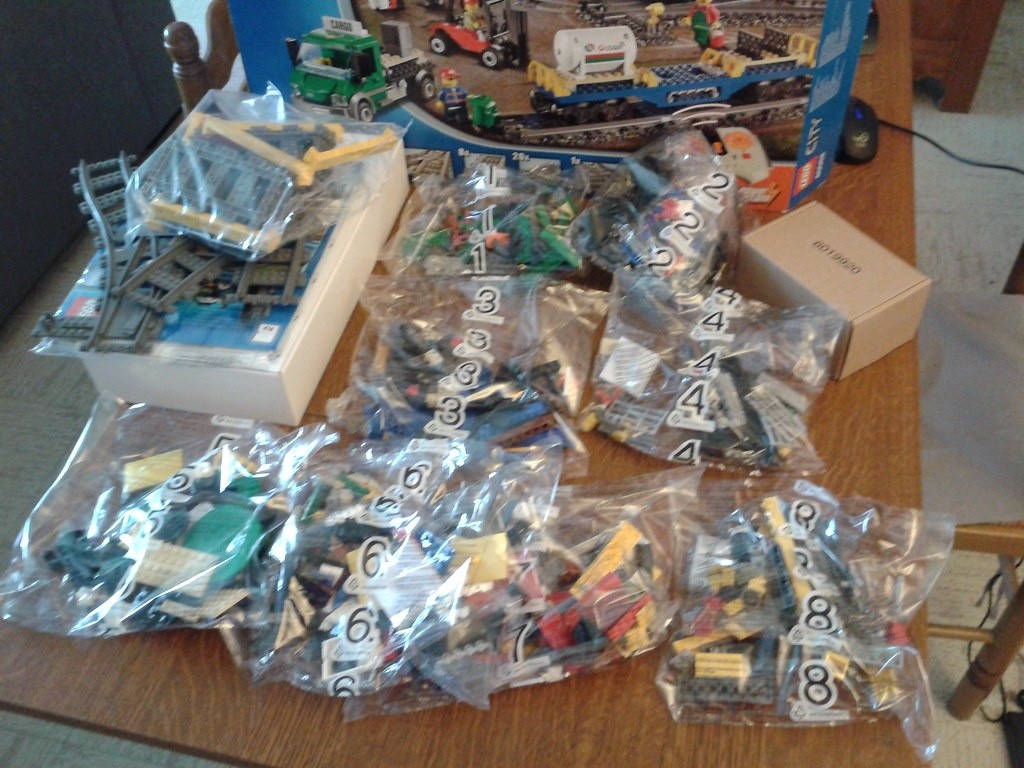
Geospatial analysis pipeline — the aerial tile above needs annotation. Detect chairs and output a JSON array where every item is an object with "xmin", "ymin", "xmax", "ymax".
[
  {"xmin": 165, "ymin": 1, "xmax": 242, "ymax": 117},
  {"xmin": 921, "ymin": 241, "xmax": 1024, "ymax": 720}
]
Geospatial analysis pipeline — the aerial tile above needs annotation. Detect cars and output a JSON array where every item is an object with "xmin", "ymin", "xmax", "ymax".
[{"xmin": 430, "ymin": 17, "xmax": 517, "ymax": 70}]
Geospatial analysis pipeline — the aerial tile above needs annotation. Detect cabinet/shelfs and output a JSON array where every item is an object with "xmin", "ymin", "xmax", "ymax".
[{"xmin": 914, "ymin": 0, "xmax": 1006, "ymax": 113}]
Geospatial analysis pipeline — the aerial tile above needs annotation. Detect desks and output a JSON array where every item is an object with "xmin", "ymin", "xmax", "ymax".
[{"xmin": 1, "ymin": 0, "xmax": 931, "ymax": 767}]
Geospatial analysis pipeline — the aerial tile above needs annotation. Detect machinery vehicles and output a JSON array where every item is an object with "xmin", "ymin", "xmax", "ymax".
[{"xmin": 294, "ymin": 15, "xmax": 436, "ymax": 123}]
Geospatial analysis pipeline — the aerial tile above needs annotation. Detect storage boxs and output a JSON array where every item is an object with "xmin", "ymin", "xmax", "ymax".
[
  {"xmin": 731, "ymin": 201, "xmax": 932, "ymax": 382},
  {"xmin": 75, "ymin": 131, "xmax": 411, "ymax": 427}
]
